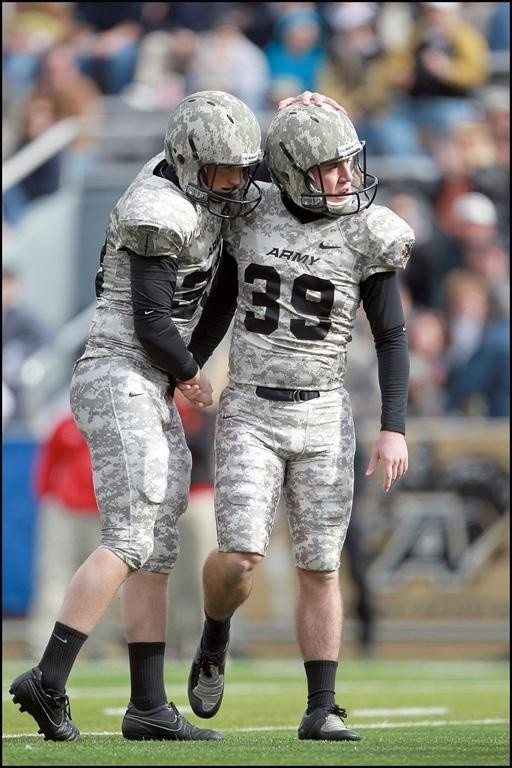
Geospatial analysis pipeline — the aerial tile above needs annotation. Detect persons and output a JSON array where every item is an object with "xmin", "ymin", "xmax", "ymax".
[
  {"xmin": 6, "ymin": 91, "xmax": 348, "ymax": 742},
  {"xmin": 171, "ymin": 100, "xmax": 416, "ymax": 741}
]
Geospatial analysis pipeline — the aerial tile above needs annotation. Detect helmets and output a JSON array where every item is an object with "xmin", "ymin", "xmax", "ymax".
[
  {"xmin": 265, "ymin": 101, "xmax": 374, "ymax": 212},
  {"xmin": 163, "ymin": 88, "xmax": 265, "ymax": 219}
]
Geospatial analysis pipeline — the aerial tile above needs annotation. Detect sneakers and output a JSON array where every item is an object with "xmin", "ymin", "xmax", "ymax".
[
  {"xmin": 8, "ymin": 666, "xmax": 81, "ymax": 742},
  {"xmin": 121, "ymin": 700, "xmax": 225, "ymax": 741},
  {"xmin": 298, "ymin": 702, "xmax": 362, "ymax": 742},
  {"xmin": 187, "ymin": 635, "xmax": 229, "ymax": 719}
]
